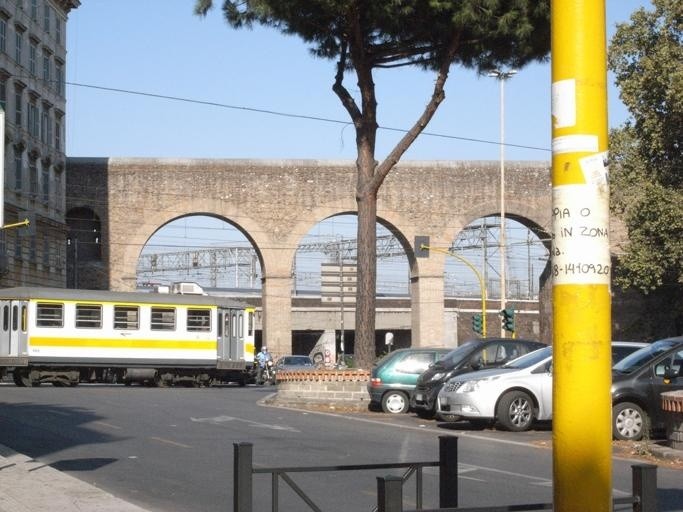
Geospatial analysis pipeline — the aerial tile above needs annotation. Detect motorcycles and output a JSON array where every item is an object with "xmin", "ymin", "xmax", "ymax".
[{"xmin": 254, "ymin": 360, "xmax": 276, "ymax": 388}]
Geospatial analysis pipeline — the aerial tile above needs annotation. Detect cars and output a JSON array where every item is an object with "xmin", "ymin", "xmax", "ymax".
[
  {"xmin": 609, "ymin": 336, "xmax": 683, "ymax": 440},
  {"xmin": 435, "ymin": 341, "xmax": 683, "ymax": 432},
  {"xmin": 270, "ymin": 355, "xmax": 313, "ymax": 373},
  {"xmin": 407, "ymin": 337, "xmax": 553, "ymax": 417},
  {"xmin": 364, "ymin": 346, "xmax": 458, "ymax": 414}
]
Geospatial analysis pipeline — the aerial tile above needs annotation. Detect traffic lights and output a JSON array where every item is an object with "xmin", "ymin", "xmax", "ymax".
[
  {"xmin": 497, "ymin": 308, "xmax": 515, "ymax": 332},
  {"xmin": 471, "ymin": 314, "xmax": 483, "ymax": 334}
]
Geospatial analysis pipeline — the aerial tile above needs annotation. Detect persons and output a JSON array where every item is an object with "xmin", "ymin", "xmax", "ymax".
[{"xmin": 255, "ymin": 345, "xmax": 272, "ymax": 384}]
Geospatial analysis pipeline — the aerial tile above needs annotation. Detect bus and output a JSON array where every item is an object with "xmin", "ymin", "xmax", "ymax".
[{"xmin": 0, "ymin": 278, "xmax": 259, "ymax": 388}]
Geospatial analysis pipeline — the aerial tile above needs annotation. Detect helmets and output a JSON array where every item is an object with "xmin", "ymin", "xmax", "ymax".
[{"xmin": 260, "ymin": 346, "xmax": 267, "ymax": 354}]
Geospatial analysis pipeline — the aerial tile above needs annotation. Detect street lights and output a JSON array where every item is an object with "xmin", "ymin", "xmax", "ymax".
[{"xmin": 485, "ymin": 68, "xmax": 517, "ymax": 361}]
